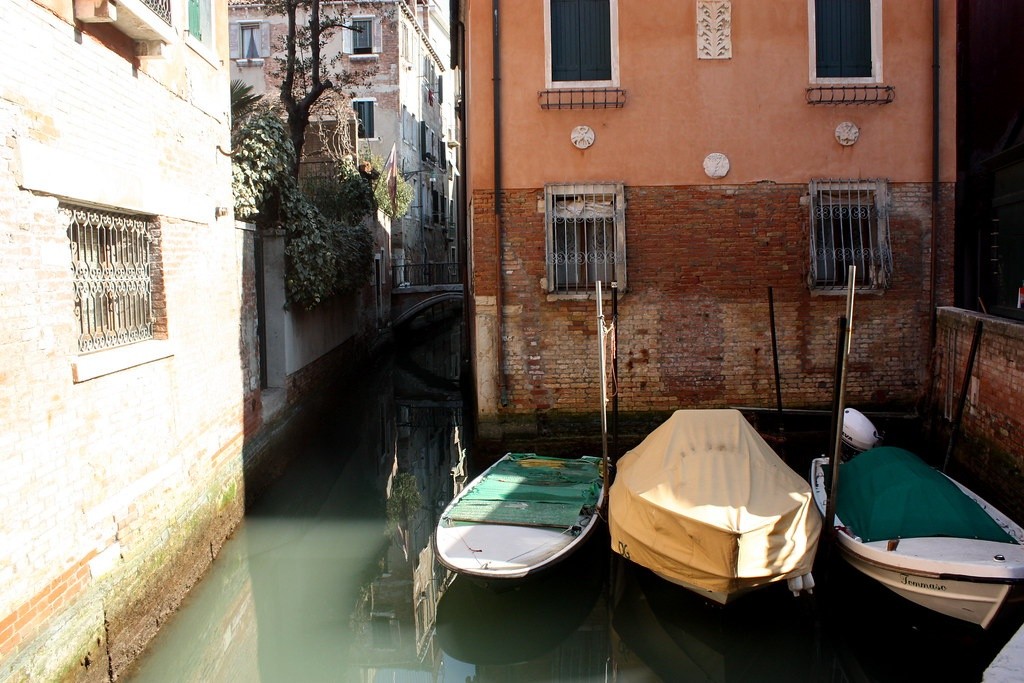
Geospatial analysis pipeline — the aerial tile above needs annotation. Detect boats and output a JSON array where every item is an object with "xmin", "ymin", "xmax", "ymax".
[
  {"xmin": 810, "ymin": 408, "xmax": 1024, "ymax": 633},
  {"xmin": 608, "ymin": 410, "xmax": 825, "ymax": 608},
  {"xmin": 433, "ymin": 450, "xmax": 608, "ymax": 591}
]
